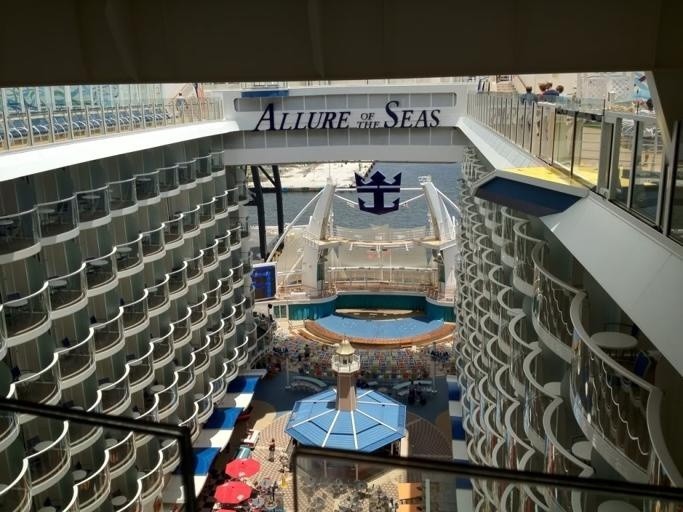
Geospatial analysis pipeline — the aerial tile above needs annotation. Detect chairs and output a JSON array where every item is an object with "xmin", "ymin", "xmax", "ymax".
[
  {"xmin": 633, "ymin": 351, "xmax": 657, "ymax": 385},
  {"xmin": 0, "ymin": 104, "xmax": 179, "ymax": 146}
]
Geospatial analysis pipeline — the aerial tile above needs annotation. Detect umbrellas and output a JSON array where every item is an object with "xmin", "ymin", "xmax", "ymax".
[{"xmin": 213, "ymin": 457, "xmax": 261, "ymax": 512}]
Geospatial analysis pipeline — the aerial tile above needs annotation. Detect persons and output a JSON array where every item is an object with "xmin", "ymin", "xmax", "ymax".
[
  {"xmin": 518, "ymin": 81, "xmax": 567, "ymax": 136},
  {"xmin": 266, "ymin": 438, "xmax": 275, "ymax": 462},
  {"xmin": 296, "ymin": 344, "xmax": 322, "ymax": 377},
  {"xmin": 176, "ymin": 92, "xmax": 188, "ymax": 119},
  {"xmin": 408, "ymin": 380, "xmax": 426, "ymax": 406}
]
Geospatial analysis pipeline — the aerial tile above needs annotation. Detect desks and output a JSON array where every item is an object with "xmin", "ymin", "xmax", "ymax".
[{"xmin": 590, "ymin": 332, "xmax": 639, "ymax": 359}]
